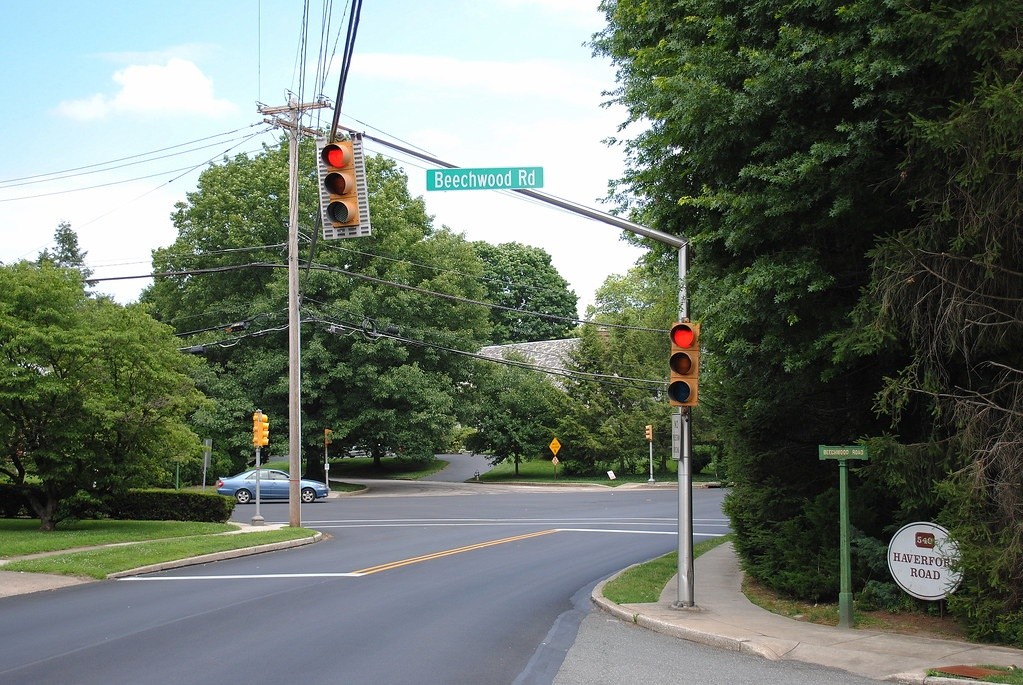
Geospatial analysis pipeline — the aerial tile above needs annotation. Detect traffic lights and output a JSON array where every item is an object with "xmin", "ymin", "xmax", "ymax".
[
  {"xmin": 256, "ymin": 415, "xmax": 269, "ymax": 447},
  {"xmin": 321, "ymin": 136, "xmax": 360, "ymax": 229},
  {"xmin": 645, "ymin": 424, "xmax": 653, "ymax": 442},
  {"xmin": 668, "ymin": 321, "xmax": 700, "ymax": 408},
  {"xmin": 325, "ymin": 430, "xmax": 333, "ymax": 444}
]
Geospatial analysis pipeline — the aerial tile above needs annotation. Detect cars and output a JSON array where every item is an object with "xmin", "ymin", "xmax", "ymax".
[{"xmin": 214, "ymin": 469, "xmax": 330, "ymax": 505}]
